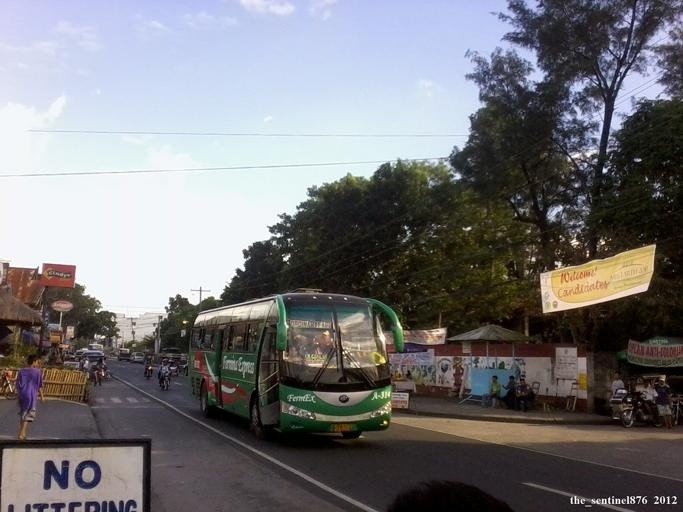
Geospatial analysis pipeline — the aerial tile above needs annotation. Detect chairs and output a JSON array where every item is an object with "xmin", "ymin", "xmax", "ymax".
[
  {"xmin": 532, "ymin": 382, "xmax": 540, "ymax": 394},
  {"xmin": 564, "ymin": 382, "xmax": 579, "ymax": 410}
]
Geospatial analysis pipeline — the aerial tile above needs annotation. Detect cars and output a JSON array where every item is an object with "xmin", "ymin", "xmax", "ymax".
[
  {"xmin": 610, "ymin": 388, "xmax": 628, "ymax": 404},
  {"xmin": 128, "ymin": 351, "xmax": 144, "ymax": 362},
  {"xmin": 73, "ymin": 343, "xmax": 106, "ymax": 372}
]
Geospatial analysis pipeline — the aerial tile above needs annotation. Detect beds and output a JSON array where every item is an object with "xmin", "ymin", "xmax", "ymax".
[{"xmin": 535, "ymin": 395, "xmax": 554, "ymax": 412}]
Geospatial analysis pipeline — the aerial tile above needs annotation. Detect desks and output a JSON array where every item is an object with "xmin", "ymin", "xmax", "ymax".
[{"xmin": 392, "ymin": 381, "xmax": 416, "ymax": 396}]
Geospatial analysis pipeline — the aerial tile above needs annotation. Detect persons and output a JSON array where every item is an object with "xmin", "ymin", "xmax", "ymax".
[
  {"xmin": 145, "ymin": 354, "xmax": 182, "ymax": 385},
  {"xmin": 80, "ymin": 356, "xmax": 109, "ymax": 385},
  {"xmin": 482, "ymin": 374, "xmax": 536, "ymax": 412},
  {"xmin": 17, "ymin": 354, "xmax": 45, "ymax": 438},
  {"xmin": 608, "ymin": 371, "xmax": 677, "ymax": 431},
  {"xmin": 387, "ymin": 480, "xmax": 513, "ymax": 511},
  {"xmin": 287, "ymin": 329, "xmax": 334, "ymax": 360}
]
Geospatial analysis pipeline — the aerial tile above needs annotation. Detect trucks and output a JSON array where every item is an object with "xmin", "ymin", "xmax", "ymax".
[{"xmin": 117, "ymin": 347, "xmax": 130, "ymax": 362}]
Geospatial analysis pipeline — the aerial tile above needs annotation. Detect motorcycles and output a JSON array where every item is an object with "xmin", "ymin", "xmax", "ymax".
[
  {"xmin": 140, "ymin": 360, "xmax": 188, "ymax": 392},
  {"xmin": 617, "ymin": 392, "xmax": 665, "ymax": 428}
]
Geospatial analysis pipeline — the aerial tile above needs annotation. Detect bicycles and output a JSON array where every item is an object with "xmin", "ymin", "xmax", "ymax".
[
  {"xmin": 0, "ymin": 367, "xmax": 17, "ymax": 401},
  {"xmin": 92, "ymin": 364, "xmax": 103, "ymax": 386}
]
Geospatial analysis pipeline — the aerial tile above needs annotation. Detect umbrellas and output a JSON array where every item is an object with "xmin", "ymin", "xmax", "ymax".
[{"xmin": 448, "ymin": 323, "xmax": 536, "ymax": 367}]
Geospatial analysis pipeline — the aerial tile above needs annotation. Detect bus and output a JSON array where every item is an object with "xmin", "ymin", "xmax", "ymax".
[{"xmin": 185, "ymin": 284, "xmax": 409, "ymax": 448}]
